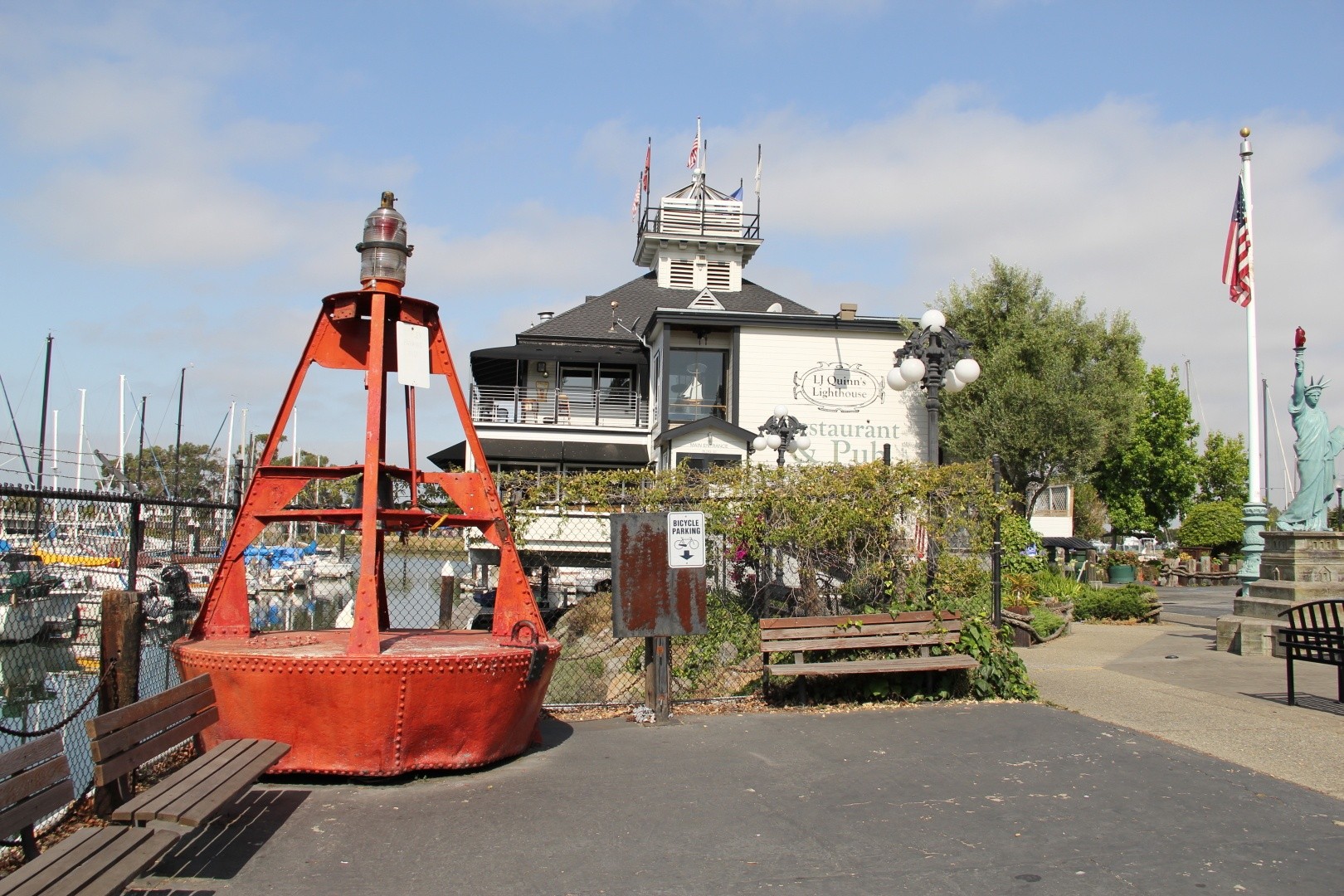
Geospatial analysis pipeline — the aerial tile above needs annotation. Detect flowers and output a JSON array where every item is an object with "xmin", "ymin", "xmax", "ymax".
[{"xmin": 1212, "ymin": 557, "xmax": 1218, "ymax": 561}]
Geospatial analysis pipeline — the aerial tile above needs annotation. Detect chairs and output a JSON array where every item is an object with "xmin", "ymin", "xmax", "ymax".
[{"xmin": 496, "ymin": 409, "xmax": 509, "ymax": 422}]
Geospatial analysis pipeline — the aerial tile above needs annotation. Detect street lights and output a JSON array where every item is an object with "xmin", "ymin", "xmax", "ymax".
[
  {"xmin": 886, "ymin": 307, "xmax": 981, "ymax": 610},
  {"xmin": 751, "ymin": 406, "xmax": 812, "ymax": 600}
]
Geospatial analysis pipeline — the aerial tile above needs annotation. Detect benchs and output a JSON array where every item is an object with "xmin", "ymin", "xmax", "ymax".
[
  {"xmin": 86, "ymin": 673, "xmax": 290, "ymax": 827},
  {"xmin": 1278, "ymin": 599, "xmax": 1344, "ymax": 704},
  {"xmin": 0, "ymin": 730, "xmax": 180, "ymax": 896},
  {"xmin": 761, "ymin": 612, "xmax": 975, "ymax": 699}
]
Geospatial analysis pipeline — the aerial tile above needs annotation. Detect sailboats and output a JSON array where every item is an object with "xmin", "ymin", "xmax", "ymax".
[{"xmin": 0, "ymin": 329, "xmax": 354, "ymax": 649}]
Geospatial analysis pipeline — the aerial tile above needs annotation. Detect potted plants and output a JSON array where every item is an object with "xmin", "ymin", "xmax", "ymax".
[
  {"xmin": 1104, "ymin": 550, "xmax": 1136, "ymax": 582},
  {"xmin": 1202, "ymin": 552, "xmax": 1239, "ymax": 571}
]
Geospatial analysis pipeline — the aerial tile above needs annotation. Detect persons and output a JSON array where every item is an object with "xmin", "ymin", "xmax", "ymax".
[{"xmin": 1274, "ymin": 353, "xmax": 1334, "ymax": 532}]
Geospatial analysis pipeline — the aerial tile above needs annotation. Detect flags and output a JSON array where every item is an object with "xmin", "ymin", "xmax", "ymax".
[
  {"xmin": 687, "ymin": 132, "xmax": 700, "ymax": 170},
  {"xmin": 632, "ymin": 179, "xmax": 640, "ymax": 224},
  {"xmin": 1219, "ymin": 171, "xmax": 1254, "ymax": 308},
  {"xmin": 725, "ymin": 186, "xmax": 743, "ymax": 200},
  {"xmin": 754, "ymin": 156, "xmax": 763, "ymax": 192},
  {"xmin": 643, "ymin": 145, "xmax": 651, "ymax": 192}
]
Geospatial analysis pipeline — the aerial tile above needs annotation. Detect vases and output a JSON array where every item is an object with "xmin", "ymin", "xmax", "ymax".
[{"xmin": 1213, "ymin": 565, "xmax": 1220, "ymax": 571}]
